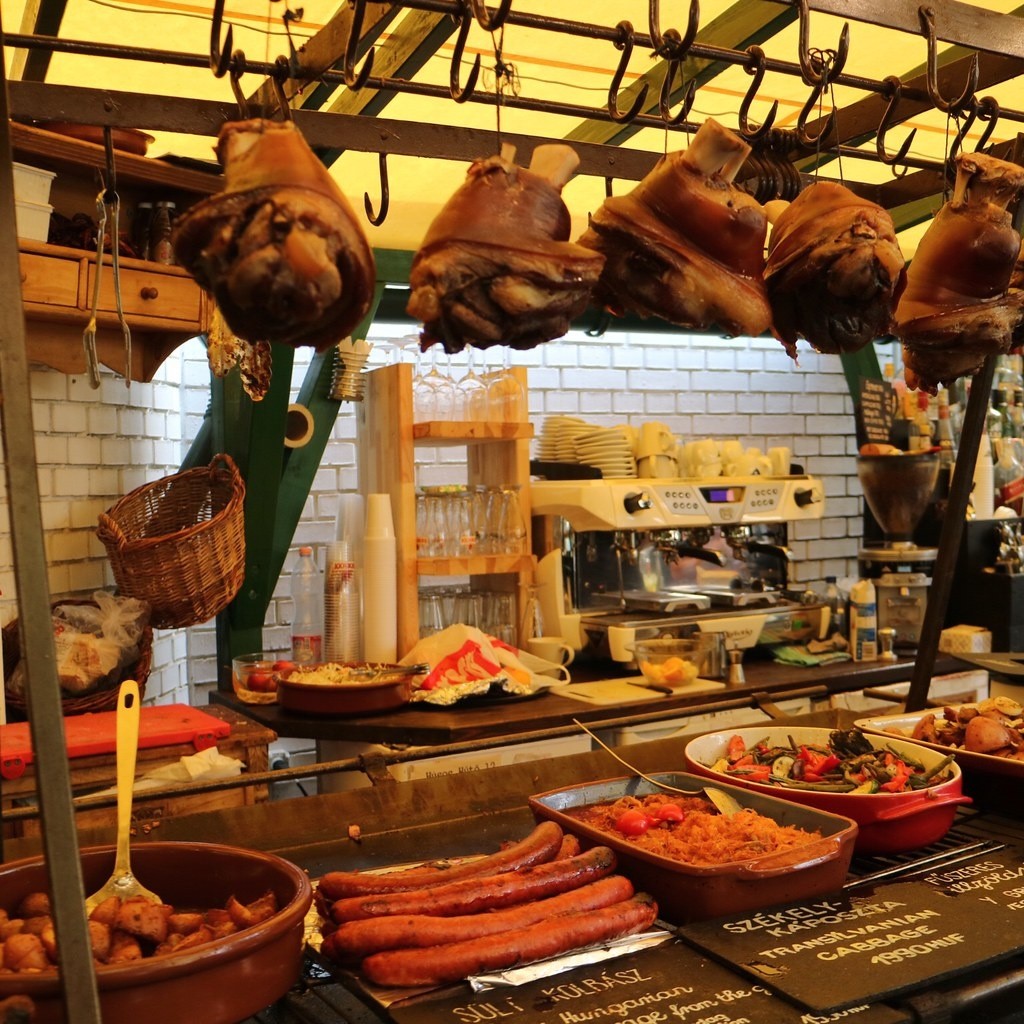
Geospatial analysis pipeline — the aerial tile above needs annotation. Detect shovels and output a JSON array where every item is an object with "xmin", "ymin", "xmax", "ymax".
[
  {"xmin": 83, "ymin": 680, "xmax": 162, "ymax": 919},
  {"xmin": 349, "ymin": 662, "xmax": 431, "ymax": 675}
]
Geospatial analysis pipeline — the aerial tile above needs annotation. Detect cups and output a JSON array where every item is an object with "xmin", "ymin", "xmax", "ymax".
[
  {"xmin": 415, "ymin": 485, "xmax": 527, "ymax": 650},
  {"xmin": 527, "ymin": 639, "xmax": 575, "ymax": 679},
  {"xmin": 693, "ymin": 631, "xmax": 728, "ymax": 679},
  {"xmin": 323, "ymin": 492, "xmax": 398, "ymax": 665},
  {"xmin": 611, "ymin": 423, "xmax": 792, "ymax": 480}
]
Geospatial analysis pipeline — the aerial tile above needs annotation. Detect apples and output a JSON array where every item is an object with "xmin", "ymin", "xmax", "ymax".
[{"xmin": 247, "ymin": 660, "xmax": 296, "ymax": 692}]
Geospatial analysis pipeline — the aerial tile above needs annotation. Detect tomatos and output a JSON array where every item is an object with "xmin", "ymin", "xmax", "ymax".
[
  {"xmin": 616, "ymin": 803, "xmax": 685, "ymax": 837},
  {"xmin": 724, "ymin": 736, "xmax": 914, "ymax": 794}
]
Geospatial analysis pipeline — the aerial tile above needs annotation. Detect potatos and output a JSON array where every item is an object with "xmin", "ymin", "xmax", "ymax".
[
  {"xmin": 0, "ymin": 890, "xmax": 277, "ymax": 977},
  {"xmin": 883, "ymin": 696, "xmax": 1024, "ymax": 764}
]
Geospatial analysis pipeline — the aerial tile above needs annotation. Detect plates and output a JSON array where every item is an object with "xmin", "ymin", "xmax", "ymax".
[
  {"xmin": 537, "ymin": 413, "xmax": 638, "ymax": 483},
  {"xmin": 853, "ymin": 704, "xmax": 1023, "ymax": 776}
]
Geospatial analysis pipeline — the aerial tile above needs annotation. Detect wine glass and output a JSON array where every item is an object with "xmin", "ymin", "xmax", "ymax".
[{"xmin": 359, "ymin": 333, "xmax": 525, "ymax": 422}]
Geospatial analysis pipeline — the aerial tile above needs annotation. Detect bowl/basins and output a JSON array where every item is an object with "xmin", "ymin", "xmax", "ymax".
[
  {"xmin": 527, "ymin": 771, "xmax": 858, "ymax": 921},
  {"xmin": 624, "ymin": 639, "xmax": 715, "ymax": 688},
  {"xmin": 1, "ymin": 841, "xmax": 312, "ymax": 1024},
  {"xmin": 232, "ymin": 651, "xmax": 313, "ymax": 705},
  {"xmin": 272, "ymin": 661, "xmax": 416, "ymax": 715},
  {"xmin": 685, "ymin": 727, "xmax": 971, "ymax": 853}
]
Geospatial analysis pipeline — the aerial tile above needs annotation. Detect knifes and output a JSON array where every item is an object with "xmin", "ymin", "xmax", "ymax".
[{"xmin": 627, "ymin": 682, "xmax": 673, "ymax": 693}]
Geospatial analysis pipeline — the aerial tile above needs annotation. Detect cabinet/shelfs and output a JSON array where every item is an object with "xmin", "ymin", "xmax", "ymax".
[
  {"xmin": 354, "ymin": 364, "xmax": 538, "ymax": 661},
  {"xmin": 863, "ymin": 358, "xmax": 1024, "ymax": 653},
  {"xmin": 8, "ymin": 112, "xmax": 213, "ymax": 382}
]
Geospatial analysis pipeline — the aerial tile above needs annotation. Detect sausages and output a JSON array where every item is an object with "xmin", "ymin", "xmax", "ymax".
[{"xmin": 315, "ymin": 821, "xmax": 658, "ymax": 987}]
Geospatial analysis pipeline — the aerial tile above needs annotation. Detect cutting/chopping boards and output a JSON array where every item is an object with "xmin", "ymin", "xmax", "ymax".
[{"xmin": 547, "ymin": 677, "xmax": 726, "ymax": 705}]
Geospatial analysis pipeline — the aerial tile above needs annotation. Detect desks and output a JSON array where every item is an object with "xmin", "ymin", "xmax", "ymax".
[
  {"xmin": 0, "ymin": 703, "xmax": 282, "ymax": 846},
  {"xmin": 209, "ymin": 642, "xmax": 991, "ymax": 794}
]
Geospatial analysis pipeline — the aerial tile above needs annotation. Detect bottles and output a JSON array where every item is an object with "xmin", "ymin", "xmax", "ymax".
[
  {"xmin": 878, "ymin": 628, "xmax": 898, "ymax": 662},
  {"xmin": 879, "ymin": 355, "xmax": 1023, "ymax": 519},
  {"xmin": 729, "ymin": 651, "xmax": 746, "ymax": 684},
  {"xmin": 290, "ymin": 548, "xmax": 322, "ymax": 666},
  {"xmin": 821, "ymin": 577, "xmax": 846, "ymax": 644},
  {"xmin": 132, "ymin": 201, "xmax": 180, "ymax": 268}
]
